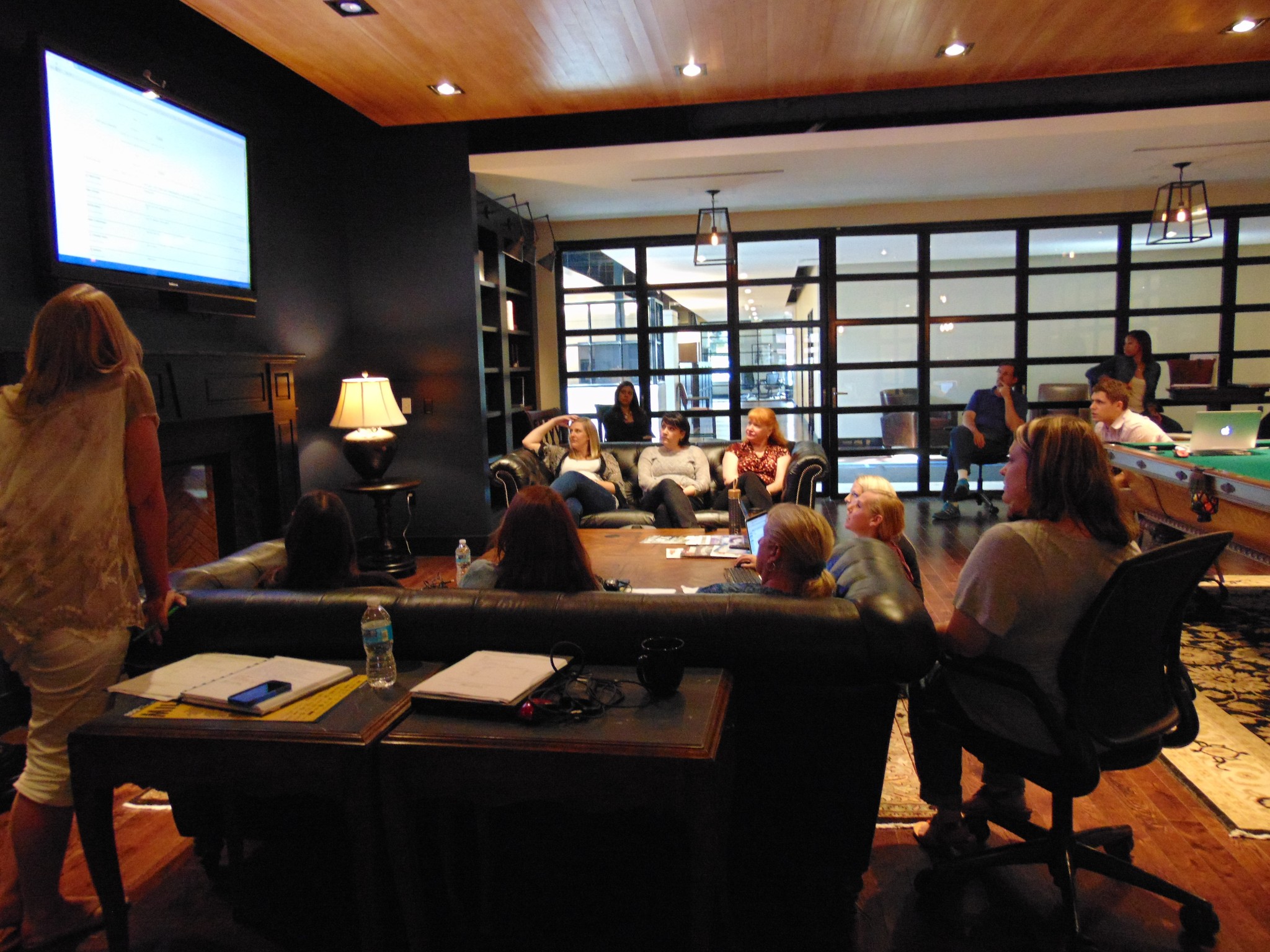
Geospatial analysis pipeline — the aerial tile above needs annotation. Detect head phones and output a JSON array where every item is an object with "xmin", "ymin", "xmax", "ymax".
[{"xmin": 520, "ymin": 689, "xmax": 575, "ymax": 724}]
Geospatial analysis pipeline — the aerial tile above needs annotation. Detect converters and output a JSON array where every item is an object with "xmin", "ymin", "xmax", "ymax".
[{"xmin": 561, "ymin": 677, "xmax": 597, "ymax": 705}]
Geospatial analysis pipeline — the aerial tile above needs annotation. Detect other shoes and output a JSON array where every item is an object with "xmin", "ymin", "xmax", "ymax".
[
  {"xmin": 932, "ymin": 503, "xmax": 961, "ymax": 519},
  {"xmin": 954, "ymin": 479, "xmax": 969, "ymax": 495},
  {"xmin": 912, "ymin": 790, "xmax": 1032, "ymax": 848}
]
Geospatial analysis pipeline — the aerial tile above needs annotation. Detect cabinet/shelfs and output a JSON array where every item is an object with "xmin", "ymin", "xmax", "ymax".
[{"xmin": 469, "ymin": 221, "xmax": 545, "ymax": 500}]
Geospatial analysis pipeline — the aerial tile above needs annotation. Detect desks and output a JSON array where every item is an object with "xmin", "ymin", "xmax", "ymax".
[
  {"xmin": 367, "ymin": 657, "xmax": 738, "ymax": 947},
  {"xmin": 68, "ymin": 658, "xmax": 452, "ymax": 952},
  {"xmin": 321, "ymin": 479, "xmax": 430, "ymax": 578}
]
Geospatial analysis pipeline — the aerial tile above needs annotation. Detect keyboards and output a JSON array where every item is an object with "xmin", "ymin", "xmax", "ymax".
[{"xmin": 723, "ymin": 566, "xmax": 763, "ymax": 585}]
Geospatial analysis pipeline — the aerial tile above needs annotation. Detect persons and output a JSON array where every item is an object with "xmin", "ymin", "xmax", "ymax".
[
  {"xmin": 601, "ymin": 380, "xmax": 653, "ymax": 441},
  {"xmin": 1088, "ymin": 376, "xmax": 1178, "ymax": 443},
  {"xmin": 637, "ymin": 411, "xmax": 712, "ymax": 529},
  {"xmin": 0, "ymin": 282, "xmax": 187, "ymax": 950},
  {"xmin": 253, "ymin": 491, "xmax": 402, "ymax": 589},
  {"xmin": 523, "ymin": 414, "xmax": 627, "ymax": 527},
  {"xmin": 908, "ymin": 414, "xmax": 1143, "ymax": 849},
  {"xmin": 720, "ymin": 408, "xmax": 789, "ymax": 515},
  {"xmin": 694, "ymin": 503, "xmax": 841, "ymax": 597},
  {"xmin": 930, "ymin": 361, "xmax": 1028, "ymax": 519},
  {"xmin": 735, "ymin": 476, "xmax": 925, "ymax": 606},
  {"xmin": 1087, "ymin": 330, "xmax": 1183, "ymax": 432},
  {"xmin": 461, "ymin": 484, "xmax": 601, "ymax": 590}
]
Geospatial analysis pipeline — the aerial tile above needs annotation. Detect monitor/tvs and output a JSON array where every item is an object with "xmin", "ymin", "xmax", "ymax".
[
  {"xmin": 34, "ymin": 33, "xmax": 260, "ymax": 302},
  {"xmin": 745, "ymin": 509, "xmax": 769, "ymax": 558}
]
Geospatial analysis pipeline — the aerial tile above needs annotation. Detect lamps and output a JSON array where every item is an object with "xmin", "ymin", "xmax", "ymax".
[
  {"xmin": 1143, "ymin": 156, "xmax": 1214, "ymax": 242},
  {"xmin": 328, "ymin": 372, "xmax": 409, "ymax": 484},
  {"xmin": 690, "ymin": 186, "xmax": 744, "ymax": 268}
]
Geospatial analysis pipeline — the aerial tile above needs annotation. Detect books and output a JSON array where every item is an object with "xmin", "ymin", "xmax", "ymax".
[{"xmin": 102, "ymin": 652, "xmax": 356, "ymax": 717}]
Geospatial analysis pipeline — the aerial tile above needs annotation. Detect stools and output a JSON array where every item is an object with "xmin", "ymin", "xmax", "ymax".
[{"xmin": 968, "ymin": 454, "xmax": 1016, "ymax": 520}]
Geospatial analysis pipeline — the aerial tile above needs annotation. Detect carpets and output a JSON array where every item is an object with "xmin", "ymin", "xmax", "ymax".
[
  {"xmin": 1146, "ymin": 563, "xmax": 1270, "ymax": 842},
  {"xmin": 872, "ymin": 691, "xmax": 945, "ymax": 826}
]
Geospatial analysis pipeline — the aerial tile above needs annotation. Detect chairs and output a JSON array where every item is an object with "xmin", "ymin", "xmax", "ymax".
[
  {"xmin": 676, "ymin": 381, "xmax": 715, "ymax": 438},
  {"xmin": 877, "ymin": 386, "xmax": 966, "ymax": 451},
  {"xmin": 905, "ymin": 523, "xmax": 1222, "ymax": 948},
  {"xmin": 522, "ymin": 406, "xmax": 573, "ymax": 453},
  {"xmin": 1024, "ymin": 381, "xmax": 1094, "ymax": 420}
]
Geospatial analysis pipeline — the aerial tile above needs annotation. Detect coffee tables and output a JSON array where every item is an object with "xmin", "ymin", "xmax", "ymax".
[{"xmin": 459, "ymin": 523, "xmax": 771, "ymax": 590}]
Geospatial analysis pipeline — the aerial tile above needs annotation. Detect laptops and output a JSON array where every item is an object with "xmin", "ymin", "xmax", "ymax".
[
  {"xmin": 1173, "ymin": 410, "xmax": 1263, "ymax": 455},
  {"xmin": 411, "ymin": 650, "xmax": 576, "ymax": 719}
]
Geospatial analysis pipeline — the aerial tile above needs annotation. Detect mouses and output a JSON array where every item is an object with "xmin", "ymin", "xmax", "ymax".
[
  {"xmin": 1175, "ymin": 446, "xmax": 1189, "ymax": 458},
  {"xmin": 604, "ymin": 578, "xmax": 619, "ymax": 588}
]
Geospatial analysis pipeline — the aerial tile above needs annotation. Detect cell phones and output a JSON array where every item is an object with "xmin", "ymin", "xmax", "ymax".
[{"xmin": 227, "ymin": 680, "xmax": 292, "ymax": 708}]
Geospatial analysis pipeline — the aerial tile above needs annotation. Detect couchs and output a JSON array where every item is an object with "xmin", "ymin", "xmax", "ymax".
[
  {"xmin": 168, "ymin": 544, "xmax": 944, "ymax": 952},
  {"xmin": 485, "ymin": 441, "xmax": 832, "ymax": 529}
]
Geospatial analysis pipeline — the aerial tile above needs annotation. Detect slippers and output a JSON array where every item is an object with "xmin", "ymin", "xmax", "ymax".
[{"xmin": 0, "ymin": 894, "xmax": 129, "ymax": 952}]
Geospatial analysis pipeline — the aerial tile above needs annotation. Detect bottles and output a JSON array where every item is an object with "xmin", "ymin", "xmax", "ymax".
[
  {"xmin": 456, "ymin": 539, "xmax": 471, "ymax": 585},
  {"xmin": 361, "ymin": 596, "xmax": 396, "ymax": 688}
]
